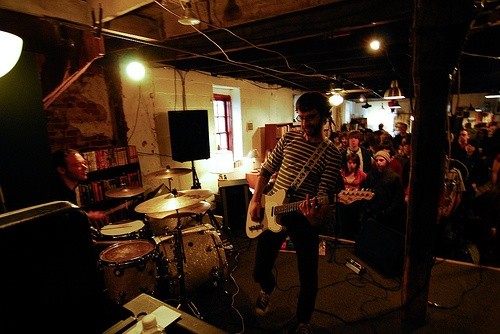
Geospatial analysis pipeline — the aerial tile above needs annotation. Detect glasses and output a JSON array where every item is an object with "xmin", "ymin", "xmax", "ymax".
[{"xmin": 296, "ymin": 112, "xmax": 320, "ymax": 122}]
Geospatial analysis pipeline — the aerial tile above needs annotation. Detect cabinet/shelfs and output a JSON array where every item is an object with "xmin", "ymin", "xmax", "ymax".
[{"xmin": 74, "ymin": 145, "xmax": 143, "ymax": 209}]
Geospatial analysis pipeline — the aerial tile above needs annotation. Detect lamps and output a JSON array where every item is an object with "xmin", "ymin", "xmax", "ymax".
[
  {"xmin": 328, "ymin": 92, "xmax": 344, "ymax": 106},
  {"xmin": 178, "ymin": 3, "xmax": 200, "ymax": 25},
  {"xmin": 390, "ymin": 100, "xmax": 402, "ymax": 109},
  {"xmin": 383, "ymin": 79, "xmax": 406, "ymax": 99}
]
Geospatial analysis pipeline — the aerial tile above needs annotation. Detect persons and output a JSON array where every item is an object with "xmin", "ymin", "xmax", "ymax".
[
  {"xmin": 250, "ymin": 92, "xmax": 342, "ymax": 334},
  {"xmin": 326, "ymin": 122, "xmax": 412, "ymax": 278},
  {"xmin": 434, "ymin": 104, "xmax": 500, "ymax": 269},
  {"xmin": 36, "ymin": 147, "xmax": 105, "ymax": 224}
]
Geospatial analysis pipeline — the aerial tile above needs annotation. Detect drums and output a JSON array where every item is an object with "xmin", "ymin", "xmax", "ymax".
[
  {"xmin": 150, "ymin": 222, "xmax": 229, "ymax": 300},
  {"xmin": 145, "ymin": 211, "xmax": 205, "ymax": 235},
  {"xmin": 103, "ymin": 242, "xmax": 158, "ymax": 304},
  {"xmin": 99, "ymin": 218, "xmax": 147, "ymax": 240}
]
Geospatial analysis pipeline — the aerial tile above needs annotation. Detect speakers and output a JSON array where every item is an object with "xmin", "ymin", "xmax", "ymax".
[{"xmin": 168, "ymin": 110, "xmax": 210, "ymax": 163}]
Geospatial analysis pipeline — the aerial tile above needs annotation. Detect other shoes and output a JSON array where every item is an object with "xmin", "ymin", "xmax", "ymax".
[
  {"xmin": 255, "ymin": 288, "xmax": 275, "ymax": 314},
  {"xmin": 295, "ymin": 323, "xmax": 312, "ymax": 334}
]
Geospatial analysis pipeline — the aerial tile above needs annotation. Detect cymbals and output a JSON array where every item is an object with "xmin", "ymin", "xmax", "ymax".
[
  {"xmin": 105, "ymin": 186, "xmax": 148, "ymax": 197},
  {"xmin": 134, "ymin": 189, "xmax": 214, "ymax": 220},
  {"xmin": 144, "ymin": 167, "xmax": 194, "ymax": 180}
]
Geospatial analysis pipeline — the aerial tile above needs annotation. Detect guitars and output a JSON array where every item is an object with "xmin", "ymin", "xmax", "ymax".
[{"xmin": 245, "ymin": 187, "xmax": 376, "ymax": 240}]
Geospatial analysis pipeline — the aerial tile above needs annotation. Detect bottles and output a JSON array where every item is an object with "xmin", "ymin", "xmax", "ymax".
[{"xmin": 138, "ymin": 314, "xmax": 167, "ymax": 334}]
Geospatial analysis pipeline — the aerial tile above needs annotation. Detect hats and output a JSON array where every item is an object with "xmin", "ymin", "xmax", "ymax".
[{"xmin": 374, "ymin": 151, "xmax": 390, "ymax": 163}]
